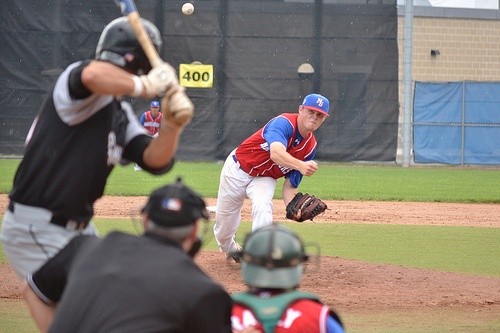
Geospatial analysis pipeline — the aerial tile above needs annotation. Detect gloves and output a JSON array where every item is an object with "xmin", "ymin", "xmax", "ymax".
[
  {"xmin": 161, "ymin": 86, "xmax": 194, "ymax": 131},
  {"xmin": 141, "ymin": 62, "xmax": 177, "ymax": 99}
]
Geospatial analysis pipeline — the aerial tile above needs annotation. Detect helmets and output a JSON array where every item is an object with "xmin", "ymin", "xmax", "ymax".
[{"xmin": 95, "ymin": 16, "xmax": 163, "ymax": 75}]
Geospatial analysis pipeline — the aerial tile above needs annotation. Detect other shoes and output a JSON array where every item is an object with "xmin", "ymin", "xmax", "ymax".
[{"xmin": 134, "ymin": 165, "xmax": 142, "ymax": 171}]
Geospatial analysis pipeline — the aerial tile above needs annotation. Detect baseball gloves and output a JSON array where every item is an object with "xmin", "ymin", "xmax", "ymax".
[{"xmin": 285, "ymin": 192, "xmax": 327, "ymax": 223}]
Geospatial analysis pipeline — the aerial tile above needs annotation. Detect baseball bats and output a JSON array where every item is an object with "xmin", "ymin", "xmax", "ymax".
[{"xmin": 113, "ymin": 0, "xmax": 189, "ymax": 127}]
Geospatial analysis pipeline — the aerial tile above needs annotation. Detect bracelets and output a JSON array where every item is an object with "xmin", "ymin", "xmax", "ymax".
[{"xmin": 129, "ymin": 74, "xmax": 143, "ymax": 99}]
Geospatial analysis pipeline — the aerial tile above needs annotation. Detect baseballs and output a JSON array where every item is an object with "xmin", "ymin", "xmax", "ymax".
[{"xmin": 181, "ymin": 3, "xmax": 194, "ymax": 15}]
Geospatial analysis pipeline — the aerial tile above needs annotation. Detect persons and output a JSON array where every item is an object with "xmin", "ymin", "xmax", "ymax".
[
  {"xmin": 229, "ymin": 226, "xmax": 348, "ymax": 333},
  {"xmin": 2, "ymin": 14, "xmax": 196, "ymax": 278},
  {"xmin": 20, "ymin": 184, "xmax": 232, "ymax": 333},
  {"xmin": 213, "ymin": 94, "xmax": 330, "ymax": 263},
  {"xmin": 134, "ymin": 101, "xmax": 162, "ymax": 172}
]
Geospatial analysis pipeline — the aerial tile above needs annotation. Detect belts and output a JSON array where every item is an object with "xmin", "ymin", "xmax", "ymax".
[
  {"xmin": 7, "ymin": 196, "xmax": 89, "ymax": 230},
  {"xmin": 232, "ymin": 155, "xmax": 249, "ymax": 173}
]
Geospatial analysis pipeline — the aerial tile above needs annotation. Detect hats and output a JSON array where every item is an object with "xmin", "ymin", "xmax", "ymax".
[
  {"xmin": 150, "ymin": 101, "xmax": 160, "ymax": 108},
  {"xmin": 239, "ymin": 224, "xmax": 305, "ymax": 288},
  {"xmin": 140, "ymin": 185, "xmax": 209, "ymax": 227},
  {"xmin": 301, "ymin": 93, "xmax": 331, "ymax": 116}
]
